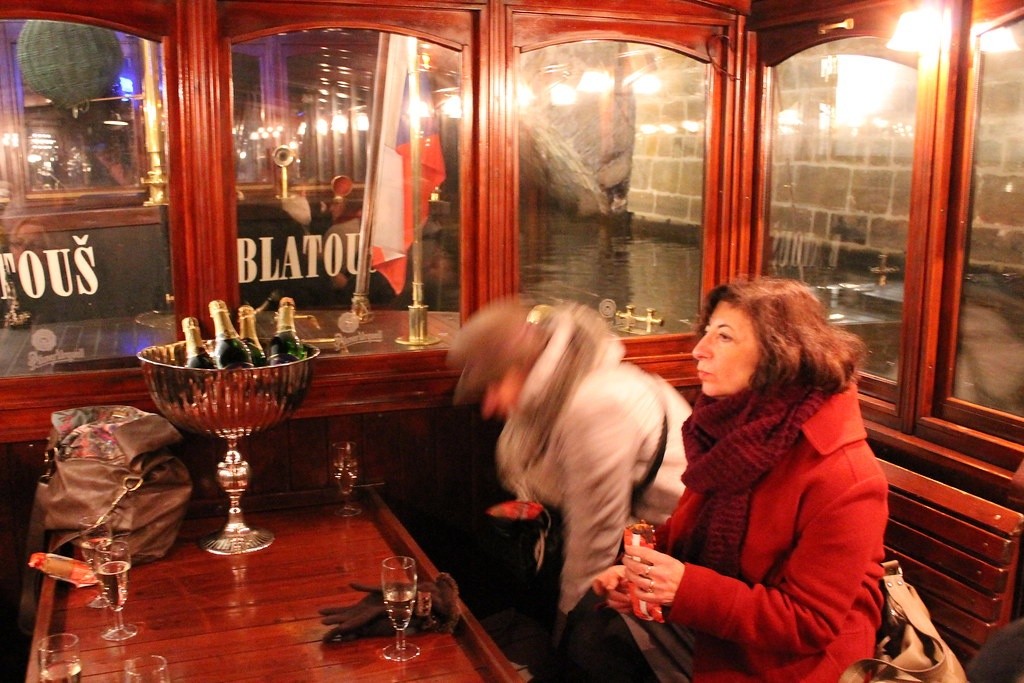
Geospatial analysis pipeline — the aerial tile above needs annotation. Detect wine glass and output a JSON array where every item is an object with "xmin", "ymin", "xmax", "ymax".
[
  {"xmin": 382, "ymin": 556, "xmax": 421, "ymax": 661},
  {"xmin": 331, "ymin": 440, "xmax": 361, "ymax": 516},
  {"xmin": 81, "ymin": 538, "xmax": 139, "ymax": 641}
]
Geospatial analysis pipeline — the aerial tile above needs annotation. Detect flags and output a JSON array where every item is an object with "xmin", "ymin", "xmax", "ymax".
[{"xmin": 370, "ymin": 32, "xmax": 448, "ymax": 295}]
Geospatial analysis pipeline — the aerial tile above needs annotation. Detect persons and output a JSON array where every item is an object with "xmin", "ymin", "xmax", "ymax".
[
  {"xmin": 543, "ymin": 279, "xmax": 889, "ymax": 683},
  {"xmin": 443, "ymin": 291, "xmax": 697, "ymax": 637}
]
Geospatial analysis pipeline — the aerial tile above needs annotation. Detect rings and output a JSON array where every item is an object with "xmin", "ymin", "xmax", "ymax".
[
  {"xmin": 646, "ymin": 567, "xmax": 651, "ymax": 576},
  {"xmin": 646, "ymin": 581, "xmax": 653, "ymax": 593}
]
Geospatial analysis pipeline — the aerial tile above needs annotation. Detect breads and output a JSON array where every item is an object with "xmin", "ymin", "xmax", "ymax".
[
  {"xmin": 626, "ymin": 523, "xmax": 654, "ymax": 544},
  {"xmin": 44, "ymin": 557, "xmax": 74, "ymax": 577}
]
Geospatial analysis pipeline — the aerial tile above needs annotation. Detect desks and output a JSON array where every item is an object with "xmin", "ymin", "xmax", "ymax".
[{"xmin": 26, "ymin": 480, "xmax": 526, "ymax": 683}]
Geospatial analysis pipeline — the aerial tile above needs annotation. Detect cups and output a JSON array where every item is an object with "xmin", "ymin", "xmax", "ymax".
[
  {"xmin": 36, "ymin": 633, "xmax": 81, "ymax": 683},
  {"xmin": 124, "ymin": 655, "xmax": 170, "ymax": 683}
]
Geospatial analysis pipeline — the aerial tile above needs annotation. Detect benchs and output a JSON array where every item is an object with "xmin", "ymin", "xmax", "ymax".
[{"xmin": 873, "ymin": 457, "xmax": 1024, "ymax": 671}]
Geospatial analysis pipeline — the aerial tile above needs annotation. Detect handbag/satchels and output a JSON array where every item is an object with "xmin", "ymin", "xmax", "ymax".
[
  {"xmin": 30, "ymin": 406, "xmax": 196, "ymax": 567},
  {"xmin": 841, "ymin": 557, "xmax": 969, "ymax": 683}
]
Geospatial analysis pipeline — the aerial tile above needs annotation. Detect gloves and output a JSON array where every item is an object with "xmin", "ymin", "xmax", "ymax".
[{"xmin": 320, "ymin": 573, "xmax": 458, "ymax": 642}]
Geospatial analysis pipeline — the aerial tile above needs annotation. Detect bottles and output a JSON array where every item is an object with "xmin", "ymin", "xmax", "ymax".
[
  {"xmin": 237, "ymin": 305, "xmax": 267, "ymax": 366},
  {"xmin": 268, "ymin": 297, "xmax": 307, "ymax": 366},
  {"xmin": 182, "ymin": 317, "xmax": 216, "ymax": 369},
  {"xmin": 208, "ymin": 299, "xmax": 254, "ymax": 369}
]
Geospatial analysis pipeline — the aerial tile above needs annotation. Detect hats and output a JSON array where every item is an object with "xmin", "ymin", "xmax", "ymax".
[{"xmin": 455, "ymin": 300, "xmax": 537, "ymax": 408}]
